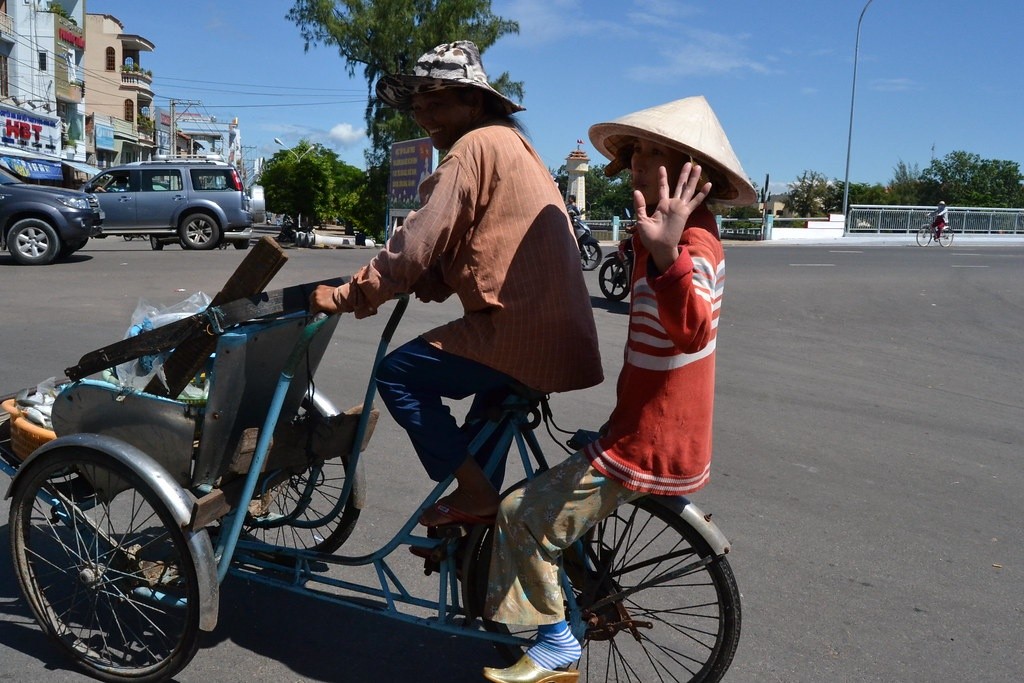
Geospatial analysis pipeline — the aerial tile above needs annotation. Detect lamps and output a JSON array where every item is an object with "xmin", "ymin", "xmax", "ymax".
[
  {"xmin": 31, "ymin": 103, "xmax": 52, "ymax": 112},
  {"xmin": 16, "ymin": 100, "xmax": 36, "ymax": 109},
  {"xmin": 0, "ymin": 95, "xmax": 20, "ymax": 106},
  {"xmin": 47, "ymin": 109, "xmax": 66, "ymax": 116}
]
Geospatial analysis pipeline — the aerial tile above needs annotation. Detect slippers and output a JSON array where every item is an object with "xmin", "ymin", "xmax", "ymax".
[
  {"xmin": 418, "ymin": 496, "xmax": 501, "ymax": 526},
  {"xmin": 483, "ymin": 653, "xmax": 579, "ymax": 683},
  {"xmin": 409, "ymin": 542, "xmax": 435, "ymax": 558}
]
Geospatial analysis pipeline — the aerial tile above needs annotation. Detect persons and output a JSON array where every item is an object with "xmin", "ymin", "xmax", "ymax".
[
  {"xmin": 483, "ymin": 95, "xmax": 758, "ymax": 682},
  {"xmin": 308, "ymin": 40, "xmax": 605, "ymax": 577},
  {"xmin": 924, "ymin": 201, "xmax": 949, "ymax": 241},
  {"xmin": 95, "ymin": 175, "xmax": 129, "ymax": 193}
]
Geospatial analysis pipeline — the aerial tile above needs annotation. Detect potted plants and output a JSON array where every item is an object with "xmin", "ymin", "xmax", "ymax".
[
  {"xmin": 119, "ymin": 61, "xmax": 153, "ymax": 85},
  {"xmin": 64, "ymin": 140, "xmax": 76, "ymax": 154},
  {"xmin": 137, "ymin": 112, "xmax": 155, "ymax": 135}
]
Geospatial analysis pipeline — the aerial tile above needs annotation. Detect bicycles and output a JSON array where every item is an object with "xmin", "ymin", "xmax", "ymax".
[{"xmin": 916, "ymin": 213, "xmax": 955, "ymax": 248}]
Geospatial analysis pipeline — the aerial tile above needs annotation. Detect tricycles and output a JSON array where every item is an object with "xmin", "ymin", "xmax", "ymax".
[{"xmin": 0, "ymin": 275, "xmax": 742, "ymax": 683}]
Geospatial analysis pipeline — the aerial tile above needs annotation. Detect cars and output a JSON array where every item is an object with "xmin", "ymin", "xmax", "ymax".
[
  {"xmin": 150, "ymin": 225, "xmax": 254, "ymax": 250},
  {"xmin": 0, "ymin": 163, "xmax": 106, "ymax": 266}
]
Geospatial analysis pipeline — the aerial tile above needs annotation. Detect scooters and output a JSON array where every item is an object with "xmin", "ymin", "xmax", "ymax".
[
  {"xmin": 565, "ymin": 205, "xmax": 603, "ymax": 272},
  {"xmin": 598, "ymin": 207, "xmax": 638, "ymax": 302}
]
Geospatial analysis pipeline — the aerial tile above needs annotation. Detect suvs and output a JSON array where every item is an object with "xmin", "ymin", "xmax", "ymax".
[{"xmin": 80, "ymin": 154, "xmax": 265, "ymax": 248}]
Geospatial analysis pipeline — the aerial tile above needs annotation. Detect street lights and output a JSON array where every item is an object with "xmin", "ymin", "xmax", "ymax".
[{"xmin": 274, "ymin": 138, "xmax": 315, "ymax": 228}]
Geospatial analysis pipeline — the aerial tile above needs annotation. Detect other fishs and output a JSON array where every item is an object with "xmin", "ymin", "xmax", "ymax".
[{"xmin": 12, "ymin": 386, "xmax": 56, "ymax": 431}]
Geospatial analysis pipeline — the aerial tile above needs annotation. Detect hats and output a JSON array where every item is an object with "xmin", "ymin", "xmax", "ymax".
[
  {"xmin": 375, "ymin": 41, "xmax": 527, "ymax": 118},
  {"xmin": 589, "ymin": 95, "xmax": 758, "ymax": 209}
]
